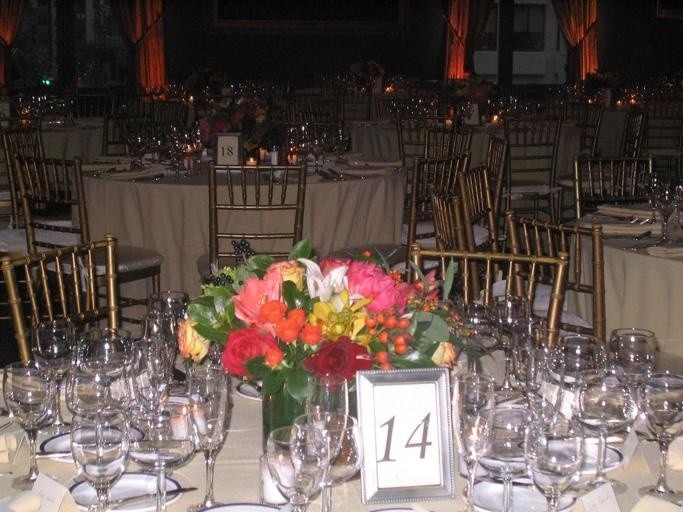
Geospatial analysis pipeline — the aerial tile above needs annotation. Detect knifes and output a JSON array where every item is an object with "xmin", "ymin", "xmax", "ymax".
[{"xmin": 90, "ymin": 486, "xmax": 198, "ymax": 512}]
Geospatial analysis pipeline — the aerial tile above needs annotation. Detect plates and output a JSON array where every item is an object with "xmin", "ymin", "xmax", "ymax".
[
  {"xmin": 199, "ymin": 504, "xmax": 279, "ymax": 512},
  {"xmin": 544, "ymin": 441, "xmax": 623, "ymax": 476},
  {"xmin": 40, "ymin": 428, "xmax": 128, "ymax": 465},
  {"xmin": 66, "ymin": 472, "xmax": 182, "ymax": 512},
  {"xmin": 467, "ymin": 470, "xmax": 575, "ymax": 512},
  {"xmin": 234, "ymin": 380, "xmax": 264, "ymax": 401}
]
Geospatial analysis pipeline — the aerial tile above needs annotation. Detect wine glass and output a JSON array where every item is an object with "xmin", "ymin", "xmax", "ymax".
[
  {"xmin": 454, "ymin": 294, "xmax": 682, "ymax": 512},
  {"xmin": 267, "ymin": 428, "xmax": 329, "ymax": 512},
  {"xmin": 642, "ymin": 174, "xmax": 682, "ymax": 245},
  {"xmin": 11, "ymin": 94, "xmax": 72, "ymax": 126},
  {"xmin": 142, "ymin": 77, "xmax": 279, "ymax": 102},
  {"xmin": 478, "ymin": 73, "xmax": 678, "ymax": 114},
  {"xmin": 0, "ymin": 289, "xmax": 231, "ymax": 512},
  {"xmin": 128, "ymin": 125, "xmax": 203, "ymax": 178},
  {"xmin": 386, "ymin": 95, "xmax": 440, "ymax": 122},
  {"xmin": 322, "ymin": 70, "xmax": 403, "ymax": 89},
  {"xmin": 302, "ymin": 373, "xmax": 348, "ymax": 512},
  {"xmin": 288, "ymin": 124, "xmax": 348, "ymax": 177},
  {"xmin": 288, "ymin": 414, "xmax": 361, "ymax": 512}
]
